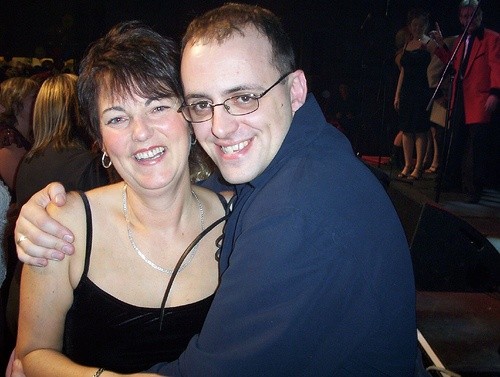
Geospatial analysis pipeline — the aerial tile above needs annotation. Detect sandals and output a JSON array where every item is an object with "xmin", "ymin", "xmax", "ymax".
[
  {"xmin": 424, "ymin": 162, "xmax": 441, "ymax": 175},
  {"xmin": 407, "ymin": 168, "xmax": 421, "ymax": 180},
  {"xmin": 397, "ymin": 163, "xmax": 414, "ymax": 178}
]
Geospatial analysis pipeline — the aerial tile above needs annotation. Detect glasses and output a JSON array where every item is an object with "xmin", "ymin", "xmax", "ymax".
[{"xmin": 177, "ymin": 70, "xmax": 296, "ymax": 124}]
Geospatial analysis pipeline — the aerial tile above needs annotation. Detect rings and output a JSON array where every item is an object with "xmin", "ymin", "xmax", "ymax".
[{"xmin": 16, "ymin": 235, "xmax": 27, "ymax": 245}]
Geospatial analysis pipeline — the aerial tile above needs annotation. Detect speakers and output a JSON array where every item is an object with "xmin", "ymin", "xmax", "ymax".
[
  {"xmin": 363, "ymin": 161, "xmax": 390, "ymax": 188},
  {"xmin": 409, "ymin": 197, "xmax": 500, "ymax": 292}
]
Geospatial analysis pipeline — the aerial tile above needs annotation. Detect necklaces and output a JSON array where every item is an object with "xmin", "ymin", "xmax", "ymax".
[{"xmin": 122, "ymin": 184, "xmax": 204, "ymax": 274}]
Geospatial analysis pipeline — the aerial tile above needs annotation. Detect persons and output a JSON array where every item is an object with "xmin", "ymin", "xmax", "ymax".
[
  {"xmin": 1, "ymin": 56, "xmax": 111, "ymax": 201},
  {"xmin": 5, "ymin": 4, "xmax": 425, "ymax": 376},
  {"xmin": 14, "ymin": 21, "xmax": 235, "ymax": 377},
  {"xmin": 393, "ymin": 0, "xmax": 500, "ymax": 192}
]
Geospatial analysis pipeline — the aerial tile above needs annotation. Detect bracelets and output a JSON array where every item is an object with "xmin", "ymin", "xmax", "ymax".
[{"xmin": 93, "ymin": 367, "xmax": 105, "ymax": 377}]
